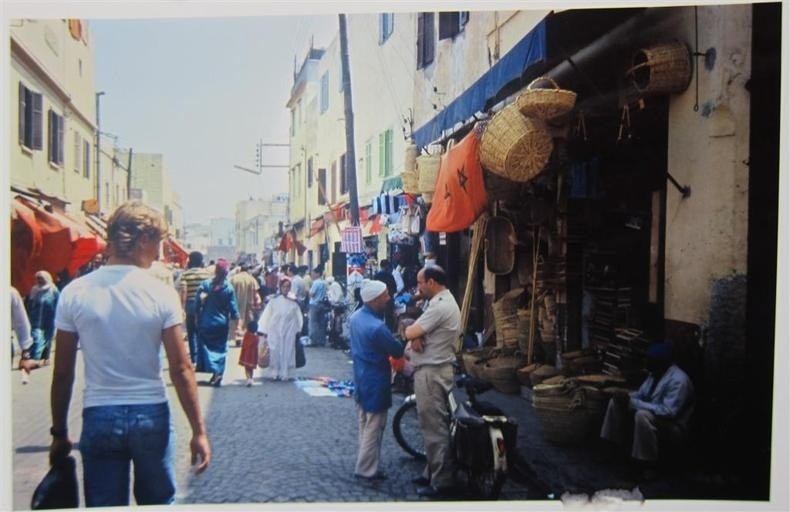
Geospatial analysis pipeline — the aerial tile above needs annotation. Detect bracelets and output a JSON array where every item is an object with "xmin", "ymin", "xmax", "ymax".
[
  {"xmin": 23, "ymin": 352, "xmax": 31, "ymax": 359},
  {"xmin": 49, "ymin": 427, "xmax": 68, "ymax": 437}
]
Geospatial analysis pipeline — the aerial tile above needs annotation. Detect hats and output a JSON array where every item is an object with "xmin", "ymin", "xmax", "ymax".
[{"xmin": 358, "ymin": 277, "xmax": 388, "ymax": 304}]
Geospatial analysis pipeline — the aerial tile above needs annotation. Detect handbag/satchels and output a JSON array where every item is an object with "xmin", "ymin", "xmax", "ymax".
[
  {"xmin": 295, "ymin": 335, "xmax": 306, "ymax": 368},
  {"xmin": 257, "ymin": 335, "xmax": 272, "ymax": 368}
]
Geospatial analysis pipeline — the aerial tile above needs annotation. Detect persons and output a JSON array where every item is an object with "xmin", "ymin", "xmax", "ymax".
[
  {"xmin": 579, "ymin": 345, "xmax": 698, "ymax": 475},
  {"xmin": 149, "ymin": 249, "xmax": 438, "ymax": 385},
  {"xmin": 349, "ymin": 278, "xmax": 407, "ymax": 484},
  {"xmin": 55, "ymin": 252, "xmax": 107, "ymax": 290},
  {"xmin": 10, "ymin": 285, "xmax": 33, "ymax": 375},
  {"xmin": 49, "ymin": 203, "xmax": 211, "ymax": 508},
  {"xmin": 26, "ymin": 270, "xmax": 61, "ymax": 367},
  {"xmin": 404, "ymin": 266, "xmax": 464, "ymax": 498}
]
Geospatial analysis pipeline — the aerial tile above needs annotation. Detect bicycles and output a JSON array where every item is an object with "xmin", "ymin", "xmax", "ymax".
[{"xmin": 393, "ymin": 369, "xmax": 514, "ymax": 501}]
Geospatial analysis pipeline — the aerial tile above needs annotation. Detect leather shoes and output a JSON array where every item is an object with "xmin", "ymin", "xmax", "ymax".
[{"xmin": 411, "ymin": 475, "xmax": 449, "ymax": 496}]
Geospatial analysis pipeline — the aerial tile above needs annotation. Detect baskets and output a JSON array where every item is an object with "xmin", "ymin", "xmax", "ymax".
[
  {"xmin": 402, "ymin": 144, "xmax": 420, "ymax": 172},
  {"xmin": 463, "ymin": 285, "xmax": 592, "ymax": 451},
  {"xmin": 414, "ymin": 152, "xmax": 444, "ymax": 194},
  {"xmin": 481, "ymin": 213, "xmax": 518, "ymax": 276},
  {"xmin": 624, "ymin": 37, "xmax": 694, "ymax": 97},
  {"xmin": 399, "ymin": 169, "xmax": 420, "ymax": 196},
  {"xmin": 514, "ymin": 75, "xmax": 580, "ymax": 121},
  {"xmin": 476, "ymin": 98, "xmax": 557, "ymax": 185}
]
